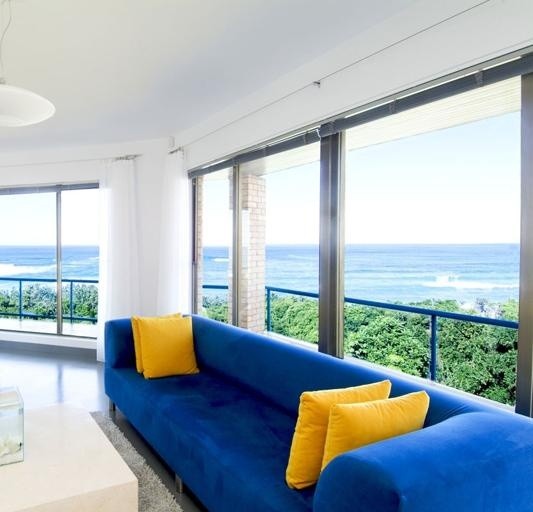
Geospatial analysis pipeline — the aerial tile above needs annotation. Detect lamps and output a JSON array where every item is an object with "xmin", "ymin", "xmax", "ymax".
[{"xmin": 1, "ymin": 0, "xmax": 58, "ymax": 130}]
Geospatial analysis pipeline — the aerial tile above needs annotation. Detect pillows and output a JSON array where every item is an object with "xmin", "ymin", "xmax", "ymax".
[
  {"xmin": 135, "ymin": 316, "xmax": 199, "ymax": 379},
  {"xmin": 319, "ymin": 388, "xmax": 429, "ymax": 472},
  {"xmin": 282, "ymin": 377, "xmax": 392, "ymax": 490},
  {"xmin": 130, "ymin": 314, "xmax": 183, "ymax": 374}
]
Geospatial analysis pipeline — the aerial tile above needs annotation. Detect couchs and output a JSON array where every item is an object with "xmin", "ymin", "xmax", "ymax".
[{"xmin": 103, "ymin": 314, "xmax": 533, "ymax": 511}]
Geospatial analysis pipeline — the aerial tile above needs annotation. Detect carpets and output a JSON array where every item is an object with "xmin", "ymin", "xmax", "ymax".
[{"xmin": 88, "ymin": 409, "xmax": 206, "ymax": 512}]
